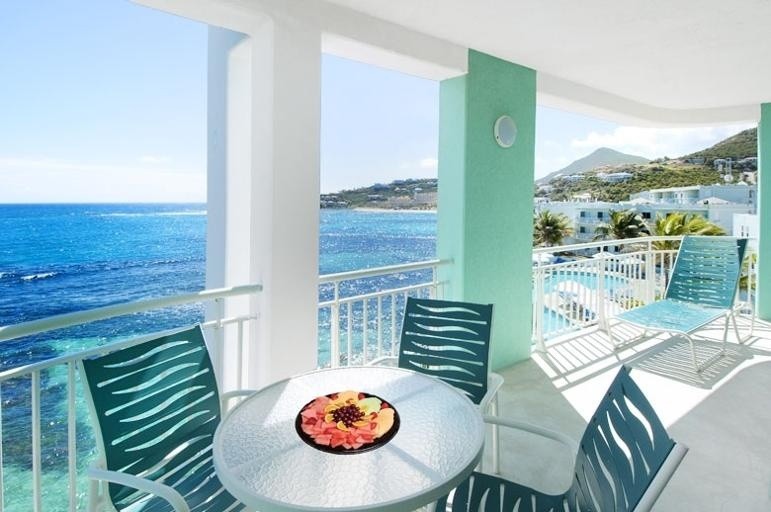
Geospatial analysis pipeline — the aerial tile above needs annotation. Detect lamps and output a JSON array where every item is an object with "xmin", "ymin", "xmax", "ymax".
[{"xmin": 493, "ymin": 115, "xmax": 518, "ymax": 148}]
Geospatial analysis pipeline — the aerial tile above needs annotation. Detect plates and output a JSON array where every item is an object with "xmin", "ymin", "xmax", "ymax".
[{"xmin": 294, "ymin": 390, "xmax": 401, "ymax": 455}]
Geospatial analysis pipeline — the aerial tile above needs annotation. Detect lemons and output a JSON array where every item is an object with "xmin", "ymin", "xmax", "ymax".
[
  {"xmin": 351, "ymin": 421, "xmax": 368, "ymax": 426},
  {"xmin": 334, "ymin": 420, "xmax": 349, "ymax": 432},
  {"xmin": 325, "ymin": 413, "xmax": 334, "ymax": 424}
]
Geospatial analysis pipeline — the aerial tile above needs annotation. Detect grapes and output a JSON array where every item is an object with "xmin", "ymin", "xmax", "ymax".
[{"xmin": 329, "ymin": 404, "xmax": 365, "ymax": 427}]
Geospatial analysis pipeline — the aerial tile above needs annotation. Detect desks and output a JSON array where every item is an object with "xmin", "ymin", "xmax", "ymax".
[{"xmin": 212, "ymin": 366, "xmax": 486, "ymax": 511}]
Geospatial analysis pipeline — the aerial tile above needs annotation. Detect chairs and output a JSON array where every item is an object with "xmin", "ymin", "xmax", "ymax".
[
  {"xmin": 431, "ymin": 365, "xmax": 689, "ymax": 512},
  {"xmin": 614, "ymin": 233, "xmax": 755, "ymax": 375},
  {"xmin": 369, "ymin": 295, "xmax": 501, "ymax": 476},
  {"xmin": 81, "ymin": 325, "xmax": 245, "ymax": 512}
]
